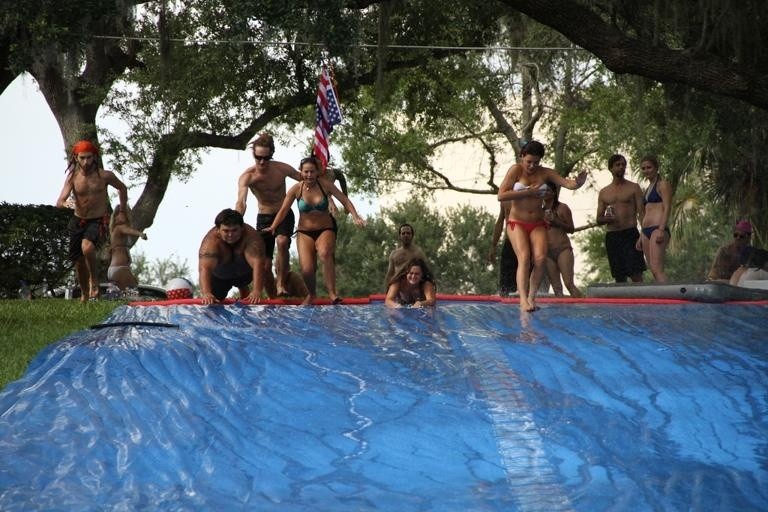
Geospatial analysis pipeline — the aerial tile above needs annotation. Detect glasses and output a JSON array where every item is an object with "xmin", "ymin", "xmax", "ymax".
[
  {"xmin": 254, "ymin": 153, "xmax": 272, "ymax": 161},
  {"xmin": 733, "ymin": 233, "xmax": 746, "ymax": 240}
]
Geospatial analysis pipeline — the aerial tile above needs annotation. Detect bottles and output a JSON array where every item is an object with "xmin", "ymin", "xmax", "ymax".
[
  {"xmin": 17, "ymin": 276, "xmax": 139, "ymax": 303},
  {"xmin": 545, "ymin": 208, "xmax": 552, "ymax": 223},
  {"xmin": 606, "ymin": 204, "xmax": 612, "ymax": 215}
]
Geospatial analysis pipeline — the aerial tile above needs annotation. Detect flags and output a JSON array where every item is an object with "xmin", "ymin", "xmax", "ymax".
[{"xmin": 309, "ymin": 55, "xmax": 342, "ymax": 175}]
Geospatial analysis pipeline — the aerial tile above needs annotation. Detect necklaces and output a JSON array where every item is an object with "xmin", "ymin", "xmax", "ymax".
[{"xmin": 303, "ymin": 183, "xmax": 315, "ymax": 191}]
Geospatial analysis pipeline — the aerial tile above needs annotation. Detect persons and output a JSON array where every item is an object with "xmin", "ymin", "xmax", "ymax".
[
  {"xmin": 105, "ymin": 204, "xmax": 148, "ymax": 289},
  {"xmin": 383, "ymin": 223, "xmax": 426, "ymax": 288},
  {"xmin": 384, "ymin": 257, "xmax": 437, "ymax": 310},
  {"xmin": 55, "ymin": 140, "xmax": 126, "ymax": 302},
  {"xmin": 489, "ymin": 198, "xmax": 534, "ymax": 297},
  {"xmin": 495, "ymin": 142, "xmax": 586, "ymax": 312},
  {"xmin": 538, "ymin": 180, "xmax": 582, "ymax": 298},
  {"xmin": 234, "ymin": 135, "xmax": 340, "ymax": 296},
  {"xmin": 311, "ymin": 147, "xmax": 351, "ymax": 215},
  {"xmin": 633, "ymin": 156, "xmax": 670, "ymax": 281},
  {"xmin": 595, "ymin": 154, "xmax": 648, "ymax": 284},
  {"xmin": 737, "ymin": 249, "xmax": 768, "ymax": 294},
  {"xmin": 707, "ymin": 220, "xmax": 755, "ymax": 280},
  {"xmin": 728, "ymin": 246, "xmax": 768, "ymax": 294},
  {"xmin": 274, "ymin": 261, "xmax": 312, "ymax": 296},
  {"xmin": 260, "ymin": 157, "xmax": 364, "ymax": 304},
  {"xmin": 197, "ymin": 208, "xmax": 269, "ymax": 306}
]
